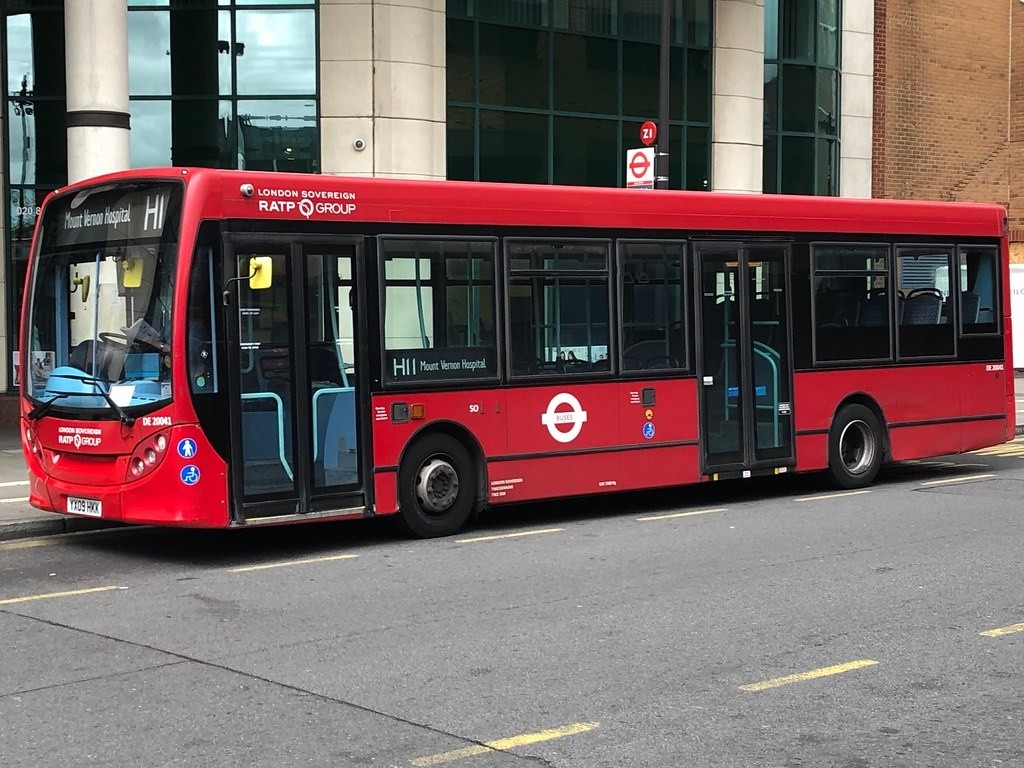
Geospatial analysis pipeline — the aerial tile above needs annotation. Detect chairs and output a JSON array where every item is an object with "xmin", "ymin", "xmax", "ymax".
[
  {"xmin": 902, "ymin": 288, "xmax": 944, "ymax": 326},
  {"xmin": 755, "ymin": 299, "xmax": 774, "ymax": 346},
  {"xmin": 947, "ymin": 290, "xmax": 981, "ymax": 324},
  {"xmin": 872, "ymin": 289, "xmax": 905, "ymax": 326},
  {"xmin": 717, "ymin": 300, "xmax": 735, "ymax": 322}
]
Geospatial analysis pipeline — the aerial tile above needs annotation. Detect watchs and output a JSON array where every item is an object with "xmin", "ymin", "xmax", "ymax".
[{"xmin": 160, "ymin": 342, "xmax": 165, "ymax": 350}]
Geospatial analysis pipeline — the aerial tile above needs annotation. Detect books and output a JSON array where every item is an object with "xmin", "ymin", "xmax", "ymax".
[{"xmin": 120, "ymin": 318, "xmax": 160, "ymax": 341}]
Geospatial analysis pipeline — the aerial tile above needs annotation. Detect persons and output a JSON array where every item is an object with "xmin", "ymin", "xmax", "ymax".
[{"xmin": 143, "ymin": 319, "xmax": 205, "ymax": 390}]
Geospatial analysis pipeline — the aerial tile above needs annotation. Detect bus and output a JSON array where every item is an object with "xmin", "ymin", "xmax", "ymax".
[{"xmin": 15, "ymin": 167, "xmax": 1020, "ymax": 542}]
